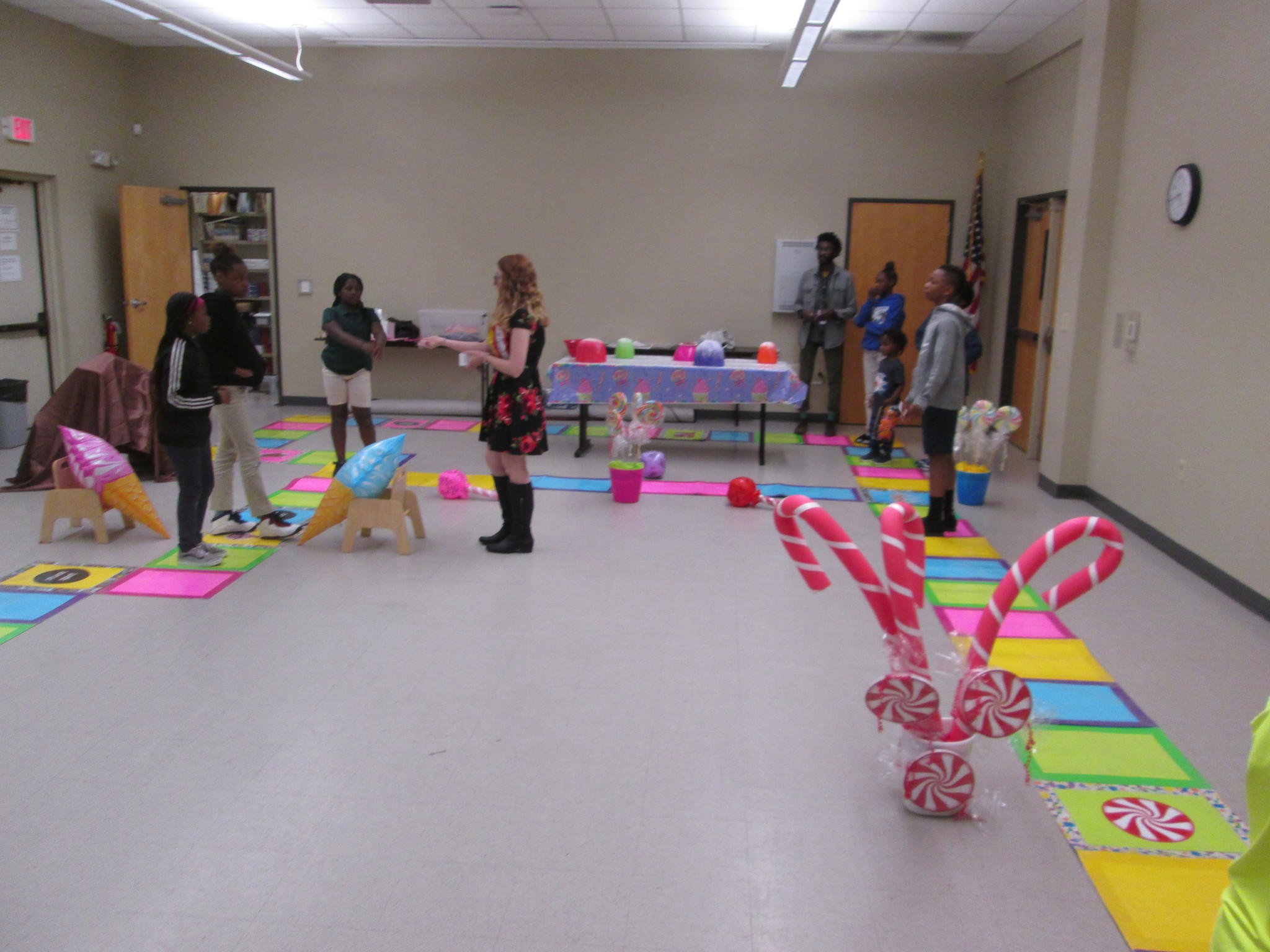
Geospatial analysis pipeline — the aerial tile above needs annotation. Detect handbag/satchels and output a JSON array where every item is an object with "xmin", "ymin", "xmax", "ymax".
[{"xmin": 913, "ymin": 316, "xmax": 985, "ymax": 366}]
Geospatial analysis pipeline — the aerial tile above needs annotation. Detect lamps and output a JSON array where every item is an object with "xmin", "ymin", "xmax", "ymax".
[
  {"xmin": 99, "ymin": 0, "xmax": 312, "ymax": 82},
  {"xmin": 777, "ymin": 0, "xmax": 842, "ymax": 90}
]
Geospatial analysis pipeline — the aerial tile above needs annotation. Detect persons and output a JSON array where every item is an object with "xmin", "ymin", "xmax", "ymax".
[
  {"xmin": 854, "ymin": 260, "xmax": 906, "ymax": 445},
  {"xmin": 794, "ymin": 232, "xmax": 857, "ymax": 437},
  {"xmin": 894, "ymin": 264, "xmax": 975, "ymax": 537},
  {"xmin": 198, "ymin": 242, "xmax": 302, "ymax": 539},
  {"xmin": 416, "ymin": 254, "xmax": 549, "ymax": 553},
  {"xmin": 322, "ymin": 273, "xmax": 386, "ymax": 478},
  {"xmin": 861, "ymin": 328, "xmax": 906, "ymax": 464},
  {"xmin": 915, "ymin": 280, "xmax": 983, "ymax": 471},
  {"xmin": 150, "ymin": 291, "xmax": 228, "ymax": 567}
]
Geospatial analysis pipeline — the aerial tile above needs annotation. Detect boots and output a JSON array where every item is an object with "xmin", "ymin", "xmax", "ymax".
[
  {"xmin": 939, "ymin": 487, "xmax": 960, "ymax": 533},
  {"xmin": 480, "ymin": 471, "xmax": 517, "ymax": 545},
  {"xmin": 910, "ymin": 493, "xmax": 951, "ymax": 539},
  {"xmin": 484, "ymin": 482, "xmax": 536, "ymax": 556}
]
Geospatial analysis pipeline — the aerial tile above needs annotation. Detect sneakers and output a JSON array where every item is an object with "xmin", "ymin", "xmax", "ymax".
[
  {"xmin": 209, "ymin": 510, "xmax": 258, "ymax": 536},
  {"xmin": 255, "ymin": 514, "xmax": 303, "ymax": 540},
  {"xmin": 824, "ymin": 418, "xmax": 838, "ymax": 437},
  {"xmin": 863, "ymin": 435, "xmax": 874, "ymax": 445},
  {"xmin": 332, "ymin": 457, "xmax": 345, "ymax": 479},
  {"xmin": 178, "ymin": 545, "xmax": 224, "ymax": 566},
  {"xmin": 856, "ymin": 434, "xmax": 867, "ymax": 443},
  {"xmin": 197, "ymin": 540, "xmax": 228, "ymax": 557},
  {"xmin": 794, "ymin": 419, "xmax": 808, "ymax": 435},
  {"xmin": 875, "ymin": 446, "xmax": 893, "ymax": 463},
  {"xmin": 858, "ymin": 448, "xmax": 880, "ymax": 461}
]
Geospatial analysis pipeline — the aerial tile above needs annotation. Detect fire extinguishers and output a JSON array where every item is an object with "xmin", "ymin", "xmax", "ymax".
[{"xmin": 101, "ymin": 313, "xmax": 119, "ymax": 356}]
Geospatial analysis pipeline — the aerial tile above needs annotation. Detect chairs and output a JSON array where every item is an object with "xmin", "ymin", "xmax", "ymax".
[
  {"xmin": 38, "ymin": 457, "xmax": 136, "ymax": 545},
  {"xmin": 341, "ymin": 465, "xmax": 426, "ymax": 555}
]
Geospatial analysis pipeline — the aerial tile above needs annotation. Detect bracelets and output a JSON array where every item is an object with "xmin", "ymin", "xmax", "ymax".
[{"xmin": 481, "ymin": 351, "xmax": 491, "ymax": 365}]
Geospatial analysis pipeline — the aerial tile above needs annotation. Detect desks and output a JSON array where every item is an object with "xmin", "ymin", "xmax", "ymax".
[
  {"xmin": 547, "ymin": 345, "xmax": 809, "ymax": 466},
  {"xmin": 314, "ymin": 337, "xmax": 490, "ymax": 413}
]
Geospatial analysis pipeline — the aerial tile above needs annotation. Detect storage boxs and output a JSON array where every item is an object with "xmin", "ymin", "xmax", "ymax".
[
  {"xmin": 206, "ymin": 223, "xmax": 269, "ymax": 271},
  {"xmin": 252, "ymin": 310, "xmax": 272, "ymax": 326},
  {"xmin": 417, "ymin": 309, "xmax": 487, "ymax": 343}
]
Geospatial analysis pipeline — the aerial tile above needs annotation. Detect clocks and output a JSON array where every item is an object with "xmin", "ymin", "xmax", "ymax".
[{"xmin": 1165, "ymin": 163, "xmax": 1201, "ymax": 226}]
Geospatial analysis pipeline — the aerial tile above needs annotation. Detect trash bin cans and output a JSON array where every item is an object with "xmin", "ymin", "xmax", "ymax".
[{"xmin": 0, "ymin": 378, "xmax": 29, "ymax": 449}]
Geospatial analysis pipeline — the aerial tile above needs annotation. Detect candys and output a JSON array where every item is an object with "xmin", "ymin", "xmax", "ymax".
[
  {"xmin": 605, "ymin": 391, "xmax": 664, "ymax": 466},
  {"xmin": 954, "ymin": 400, "xmax": 1023, "ymax": 468}
]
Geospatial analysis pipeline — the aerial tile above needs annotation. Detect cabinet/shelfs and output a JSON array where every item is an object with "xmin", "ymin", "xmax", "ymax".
[{"xmin": 194, "ymin": 211, "xmax": 273, "ymax": 374}]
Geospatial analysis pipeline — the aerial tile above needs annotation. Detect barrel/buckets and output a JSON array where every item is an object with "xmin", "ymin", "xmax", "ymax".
[
  {"xmin": 895, "ymin": 718, "xmax": 979, "ymax": 819},
  {"xmin": 609, "ymin": 461, "xmax": 644, "ymax": 503},
  {"xmin": 955, "ymin": 463, "xmax": 992, "ymax": 506}
]
